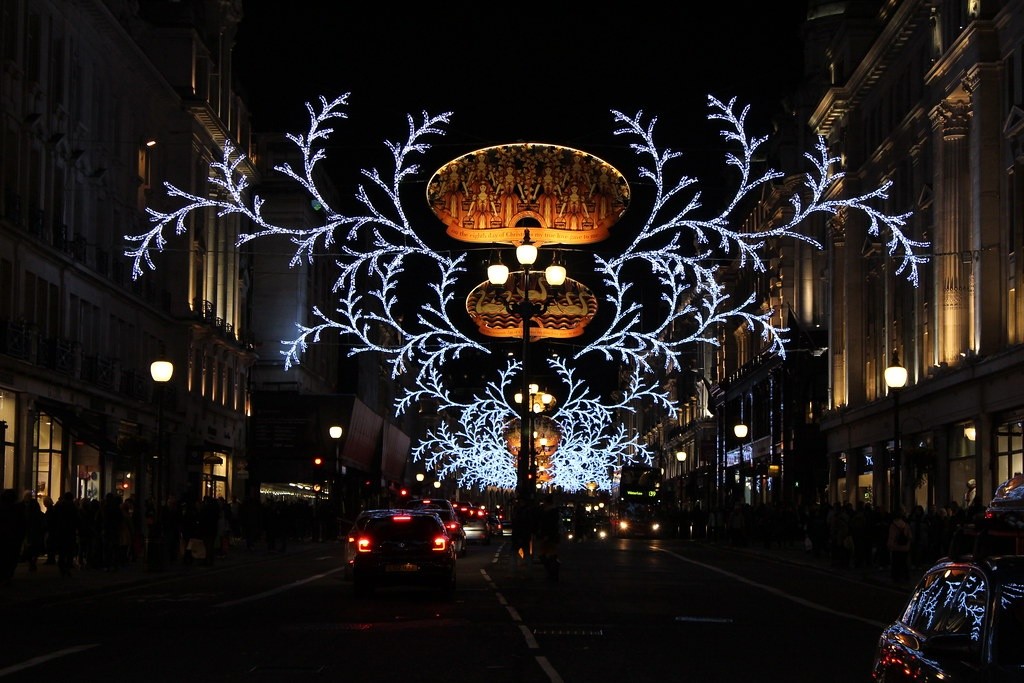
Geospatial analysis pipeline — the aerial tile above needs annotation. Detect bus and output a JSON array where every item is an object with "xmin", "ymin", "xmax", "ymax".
[{"xmin": 612, "ymin": 464, "xmax": 662, "ymax": 539}]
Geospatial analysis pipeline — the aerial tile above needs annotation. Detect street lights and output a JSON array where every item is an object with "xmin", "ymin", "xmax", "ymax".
[
  {"xmin": 328, "ymin": 425, "xmax": 343, "ymax": 538},
  {"xmin": 487, "ymin": 230, "xmax": 567, "ymax": 543},
  {"xmin": 885, "ymin": 352, "xmax": 908, "ymax": 518},
  {"xmin": 416, "ymin": 473, "xmax": 424, "ymax": 499},
  {"xmin": 149, "ymin": 360, "xmax": 175, "ymax": 557},
  {"xmin": 433, "ymin": 481, "xmax": 442, "ymax": 499},
  {"xmin": 734, "ymin": 424, "xmax": 749, "ymax": 548}
]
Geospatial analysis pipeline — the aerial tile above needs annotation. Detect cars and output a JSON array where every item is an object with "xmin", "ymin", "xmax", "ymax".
[
  {"xmin": 405, "ymin": 499, "xmax": 466, "ymax": 551},
  {"xmin": 419, "ymin": 508, "xmax": 465, "ymax": 558},
  {"xmin": 486, "ymin": 516, "xmax": 501, "ymax": 537},
  {"xmin": 583, "ymin": 517, "xmax": 610, "ymax": 540},
  {"xmin": 500, "ymin": 520, "xmax": 513, "ymax": 537},
  {"xmin": 871, "ymin": 533, "xmax": 1024, "ymax": 683},
  {"xmin": 335, "ymin": 509, "xmax": 457, "ymax": 597},
  {"xmin": 454, "ymin": 506, "xmax": 492, "ymax": 545}
]
